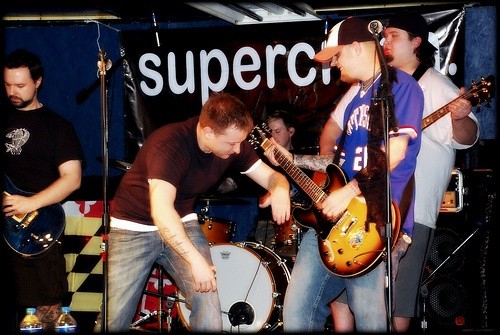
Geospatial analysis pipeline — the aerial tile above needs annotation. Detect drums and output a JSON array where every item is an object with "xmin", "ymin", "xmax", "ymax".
[
  {"xmin": 274, "ymin": 202, "xmax": 309, "ymax": 257},
  {"xmin": 175, "ymin": 242, "xmax": 291, "ymax": 332},
  {"xmin": 197, "ymin": 215, "xmax": 238, "ymax": 248}
]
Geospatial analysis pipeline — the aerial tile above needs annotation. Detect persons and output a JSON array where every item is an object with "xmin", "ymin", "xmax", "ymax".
[
  {"xmin": 263, "ymin": 16, "xmax": 424, "ymax": 332},
  {"xmin": 94, "ymin": 91, "xmax": 303, "ymax": 331},
  {"xmin": 0, "ymin": 52, "xmax": 85, "ymax": 328},
  {"xmin": 320, "ymin": 10, "xmax": 481, "ymax": 332}
]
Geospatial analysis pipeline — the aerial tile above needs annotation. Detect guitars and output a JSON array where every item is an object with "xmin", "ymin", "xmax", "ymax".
[
  {"xmin": 0, "ymin": 170, "xmax": 66, "ymax": 260},
  {"xmin": 247, "ymin": 123, "xmax": 402, "ymax": 280},
  {"xmin": 421, "ymin": 75, "xmax": 494, "ymax": 132}
]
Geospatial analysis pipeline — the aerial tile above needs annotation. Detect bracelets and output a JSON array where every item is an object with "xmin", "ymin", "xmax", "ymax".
[{"xmin": 292, "ymin": 153, "xmax": 294, "ymax": 163}]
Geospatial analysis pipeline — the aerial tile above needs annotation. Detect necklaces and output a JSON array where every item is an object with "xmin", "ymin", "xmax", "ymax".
[{"xmin": 361, "ymin": 68, "xmax": 383, "ymax": 91}]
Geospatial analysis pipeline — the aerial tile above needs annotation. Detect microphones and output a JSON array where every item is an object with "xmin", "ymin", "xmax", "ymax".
[
  {"xmin": 96, "ymin": 49, "xmax": 112, "ymax": 71},
  {"xmin": 368, "ymin": 20, "xmax": 383, "ymax": 35},
  {"xmin": 409, "ymin": 209, "xmax": 487, "ymax": 335}
]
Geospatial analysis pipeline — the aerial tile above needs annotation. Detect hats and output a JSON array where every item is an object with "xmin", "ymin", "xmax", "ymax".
[
  {"xmin": 313, "ymin": 16, "xmax": 376, "ymax": 62},
  {"xmin": 385, "ymin": 11, "xmax": 430, "ymax": 44}
]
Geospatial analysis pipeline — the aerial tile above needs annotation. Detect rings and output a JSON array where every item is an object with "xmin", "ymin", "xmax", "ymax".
[{"xmin": 11, "ymin": 206, "xmax": 14, "ymax": 211}]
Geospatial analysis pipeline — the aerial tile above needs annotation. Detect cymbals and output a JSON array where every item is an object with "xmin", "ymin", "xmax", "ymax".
[
  {"xmin": 288, "ymin": 143, "xmax": 320, "ymax": 153},
  {"xmin": 196, "ymin": 195, "xmax": 250, "ymax": 206},
  {"xmin": 96, "ymin": 156, "xmax": 134, "ymax": 172}
]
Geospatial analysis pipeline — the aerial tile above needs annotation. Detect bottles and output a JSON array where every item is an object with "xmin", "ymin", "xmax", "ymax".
[
  {"xmin": 55, "ymin": 306, "xmax": 77, "ymax": 333},
  {"xmin": 19, "ymin": 307, "xmax": 43, "ymax": 334}
]
